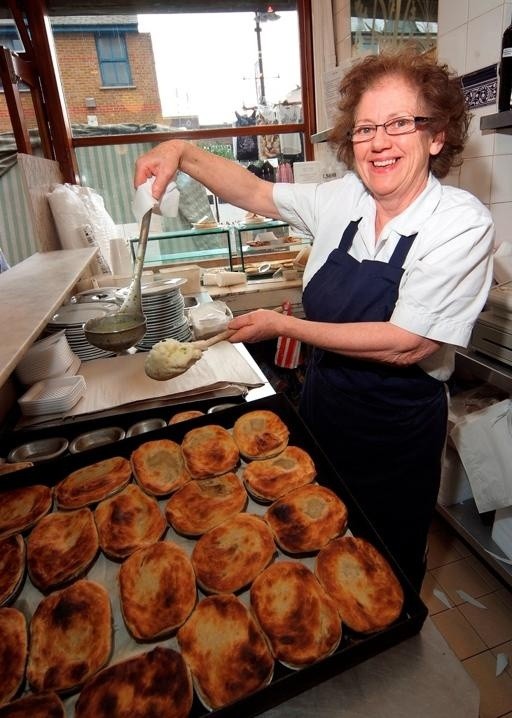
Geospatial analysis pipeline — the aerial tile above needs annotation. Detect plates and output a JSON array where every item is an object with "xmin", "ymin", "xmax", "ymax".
[
  {"xmin": 41, "ymin": 265, "xmax": 201, "ymax": 359},
  {"xmin": 13, "ymin": 329, "xmax": 89, "ymax": 414}
]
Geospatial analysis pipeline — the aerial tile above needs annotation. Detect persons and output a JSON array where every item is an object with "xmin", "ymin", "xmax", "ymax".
[{"xmin": 134, "ymin": 41, "xmax": 494, "ymax": 596}]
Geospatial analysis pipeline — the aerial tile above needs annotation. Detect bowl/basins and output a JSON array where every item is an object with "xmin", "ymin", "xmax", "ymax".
[
  {"xmin": 190, "ymin": 221, "xmax": 219, "ymax": 229},
  {"xmin": 242, "ymin": 217, "xmax": 265, "ymax": 221},
  {"xmin": 7, "ymin": 401, "xmax": 242, "ymax": 463}
]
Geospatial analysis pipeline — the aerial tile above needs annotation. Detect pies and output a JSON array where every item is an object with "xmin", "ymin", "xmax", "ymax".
[
  {"xmin": 129, "ymin": 440, "xmax": 191, "ymax": 496},
  {"xmin": 250, "ymin": 561, "xmax": 342, "ymax": 671},
  {"xmin": 180, "ymin": 425, "xmax": 240, "ymax": 479},
  {"xmin": 176, "ymin": 593, "xmax": 275, "ymax": 711},
  {"xmin": 316, "ymin": 536, "xmax": 404, "ymax": 633},
  {"xmin": 1, "ymin": 608, "xmax": 27, "ymax": 703},
  {"xmin": 119, "ymin": 541, "xmax": 198, "ymax": 641},
  {"xmin": 73, "ymin": 647, "xmax": 193, "ymax": 717},
  {"xmin": 25, "ymin": 507, "xmax": 99, "ymax": 590},
  {"xmin": 1, "ymin": 691, "xmax": 66, "ymax": 717},
  {"xmin": 242, "ymin": 446, "xmax": 316, "ymax": 502},
  {"xmin": 191, "ymin": 513, "xmax": 276, "ymax": 593},
  {"xmin": 26, "ymin": 580, "xmax": 112, "ymax": 693},
  {"xmin": 233, "ymin": 410, "xmax": 290, "ymax": 459},
  {"xmin": 0, "ymin": 534, "xmax": 26, "ymax": 607},
  {"xmin": 53, "ymin": 456, "xmax": 132, "ymax": 510},
  {"xmin": 263, "ymin": 484, "xmax": 349, "ymax": 553},
  {"xmin": 94, "ymin": 484, "xmax": 168, "ymax": 560},
  {"xmin": 1, "ymin": 485, "xmax": 53, "ymax": 540},
  {"xmin": 164, "ymin": 473, "xmax": 247, "ymax": 537}
]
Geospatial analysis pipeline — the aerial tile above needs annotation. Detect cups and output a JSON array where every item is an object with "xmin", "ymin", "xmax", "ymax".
[
  {"xmin": 108, "ymin": 238, "xmax": 132, "ymax": 274},
  {"xmin": 203, "ymin": 269, "xmax": 246, "ymax": 287}
]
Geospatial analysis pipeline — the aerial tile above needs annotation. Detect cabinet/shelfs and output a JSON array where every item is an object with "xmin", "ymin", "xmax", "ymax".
[
  {"xmin": 232, "ymin": 218, "xmax": 314, "ymax": 270},
  {"xmin": 434, "ymin": 344, "xmax": 511, "ymax": 588},
  {"xmin": 126, "ymin": 223, "xmax": 234, "ymax": 275}
]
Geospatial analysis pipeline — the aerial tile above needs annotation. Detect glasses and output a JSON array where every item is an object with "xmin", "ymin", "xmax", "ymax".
[{"xmin": 349, "ymin": 115, "xmax": 438, "ymax": 142}]
